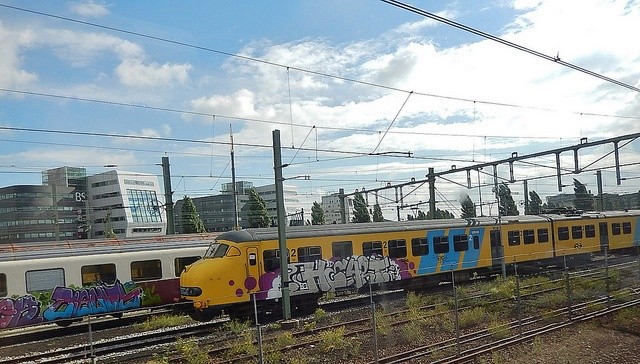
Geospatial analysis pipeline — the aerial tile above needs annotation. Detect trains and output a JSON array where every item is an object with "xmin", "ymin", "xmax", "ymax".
[
  {"xmin": 0, "ymin": 231, "xmax": 224, "ymax": 333},
  {"xmin": 179, "ymin": 208, "xmax": 640, "ymax": 321}
]
